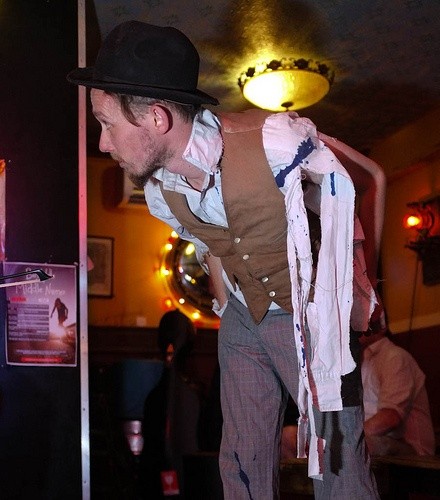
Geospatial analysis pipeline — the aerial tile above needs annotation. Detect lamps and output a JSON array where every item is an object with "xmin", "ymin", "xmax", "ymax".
[{"xmin": 238, "ymin": 58, "xmax": 335, "ymax": 112}]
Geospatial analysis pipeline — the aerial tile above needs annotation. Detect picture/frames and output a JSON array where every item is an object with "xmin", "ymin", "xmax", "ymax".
[{"xmin": 87, "ymin": 235, "xmax": 115, "ymax": 299}]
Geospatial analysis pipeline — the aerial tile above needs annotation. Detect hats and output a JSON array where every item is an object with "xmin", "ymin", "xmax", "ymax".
[{"xmin": 66, "ymin": 20, "xmax": 219, "ymax": 105}]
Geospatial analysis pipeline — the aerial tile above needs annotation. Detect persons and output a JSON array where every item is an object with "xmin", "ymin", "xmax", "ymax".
[
  {"xmin": 138, "ymin": 307, "xmax": 221, "ymax": 500},
  {"xmin": 357, "ymin": 319, "xmax": 439, "ymax": 500},
  {"xmin": 49, "ymin": 298, "xmax": 69, "ymax": 329},
  {"xmin": 67, "ymin": 22, "xmax": 387, "ymax": 500}
]
